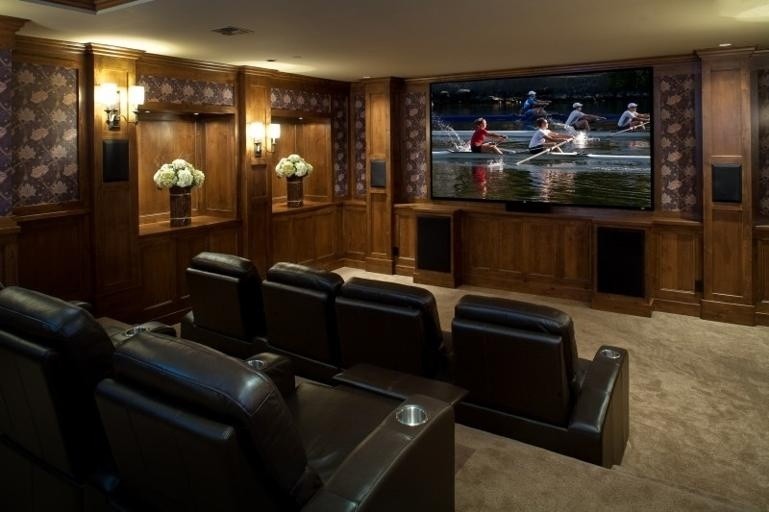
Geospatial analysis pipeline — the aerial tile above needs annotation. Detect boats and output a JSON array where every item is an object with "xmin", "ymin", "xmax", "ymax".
[
  {"xmin": 434, "ymin": 146, "xmax": 651, "ymax": 172},
  {"xmin": 432, "ymin": 130, "xmax": 651, "ymax": 149}
]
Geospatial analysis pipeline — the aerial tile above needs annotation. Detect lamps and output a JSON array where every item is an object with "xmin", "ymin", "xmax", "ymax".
[
  {"xmin": 251, "ymin": 121, "xmax": 264, "ymax": 157},
  {"xmin": 132, "ymin": 85, "xmax": 152, "ymax": 126},
  {"xmin": 264, "ymin": 123, "xmax": 281, "ymax": 155},
  {"xmin": 99, "ymin": 81, "xmax": 122, "ymax": 129}
]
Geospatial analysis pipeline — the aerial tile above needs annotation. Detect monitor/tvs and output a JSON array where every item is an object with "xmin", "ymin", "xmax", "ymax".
[{"xmin": 428, "ymin": 66, "xmax": 654, "ymax": 214}]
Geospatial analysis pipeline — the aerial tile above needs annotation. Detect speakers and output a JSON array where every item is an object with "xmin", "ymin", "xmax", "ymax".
[
  {"xmin": 416, "ymin": 216, "xmax": 451, "ymax": 274},
  {"xmin": 596, "ymin": 226, "xmax": 645, "ymax": 298},
  {"xmin": 711, "ymin": 164, "xmax": 742, "ymax": 203},
  {"xmin": 101, "ymin": 139, "xmax": 128, "ymax": 183},
  {"xmin": 369, "ymin": 160, "xmax": 385, "ymax": 187}
]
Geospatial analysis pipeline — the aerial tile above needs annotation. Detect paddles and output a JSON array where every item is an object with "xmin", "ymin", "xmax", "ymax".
[
  {"xmin": 517, "ymin": 138, "xmax": 573, "ymax": 164},
  {"xmin": 553, "ymin": 119, "xmax": 604, "ymax": 128},
  {"xmin": 616, "ymin": 122, "xmax": 651, "ymax": 135},
  {"xmin": 496, "ymin": 137, "xmax": 508, "ymax": 146}
]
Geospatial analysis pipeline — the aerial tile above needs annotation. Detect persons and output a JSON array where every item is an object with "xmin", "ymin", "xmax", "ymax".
[
  {"xmin": 565, "ymin": 102, "xmax": 607, "ymax": 131},
  {"xmin": 528, "ymin": 118, "xmax": 567, "ymax": 155},
  {"xmin": 521, "ymin": 90, "xmax": 552, "ymax": 119},
  {"xmin": 471, "ymin": 161, "xmax": 488, "ymax": 199},
  {"xmin": 617, "ymin": 103, "xmax": 650, "ymax": 130},
  {"xmin": 471, "ymin": 117, "xmax": 507, "ymax": 155}
]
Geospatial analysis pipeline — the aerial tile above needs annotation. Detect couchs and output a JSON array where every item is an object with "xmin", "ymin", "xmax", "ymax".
[
  {"xmin": 0, "ymin": 286, "xmax": 177, "ymax": 512},
  {"xmin": 90, "ymin": 326, "xmax": 456, "ymax": 512},
  {"xmin": 334, "ymin": 275, "xmax": 456, "ymax": 384},
  {"xmin": 257, "ymin": 261, "xmax": 345, "ymax": 383},
  {"xmin": 450, "ymin": 292, "xmax": 632, "ymax": 470},
  {"xmin": 183, "ymin": 250, "xmax": 258, "ymax": 354}
]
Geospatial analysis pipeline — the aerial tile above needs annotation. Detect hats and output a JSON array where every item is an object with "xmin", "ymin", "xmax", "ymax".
[
  {"xmin": 572, "ymin": 103, "xmax": 583, "ymax": 108},
  {"xmin": 528, "ymin": 90, "xmax": 537, "ymax": 95},
  {"xmin": 628, "ymin": 102, "xmax": 639, "ymax": 109}
]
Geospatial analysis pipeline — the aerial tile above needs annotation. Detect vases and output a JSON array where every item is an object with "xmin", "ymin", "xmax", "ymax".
[
  {"xmin": 286, "ymin": 175, "xmax": 304, "ymax": 208},
  {"xmin": 168, "ymin": 184, "xmax": 192, "ymax": 227}
]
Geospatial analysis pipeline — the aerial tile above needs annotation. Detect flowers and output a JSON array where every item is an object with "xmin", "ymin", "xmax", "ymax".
[
  {"xmin": 154, "ymin": 159, "xmax": 206, "ymax": 190},
  {"xmin": 274, "ymin": 153, "xmax": 313, "ymax": 181}
]
